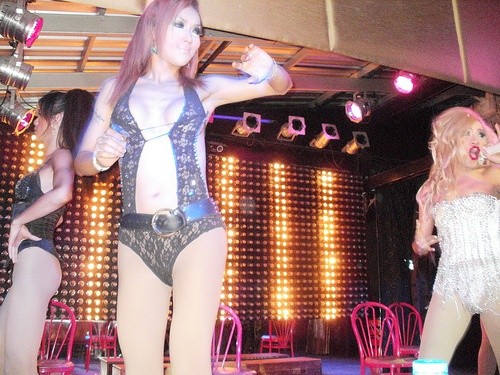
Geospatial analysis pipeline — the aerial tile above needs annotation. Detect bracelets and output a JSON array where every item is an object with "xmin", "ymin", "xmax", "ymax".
[
  {"xmin": 267, "ymin": 58, "xmax": 278, "ymax": 80},
  {"xmin": 92, "ymin": 152, "xmax": 109, "ymax": 171}
]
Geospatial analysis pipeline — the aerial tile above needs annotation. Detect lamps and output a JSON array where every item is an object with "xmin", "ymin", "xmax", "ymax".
[
  {"xmin": 345, "ymin": 98, "xmax": 378, "ymax": 123},
  {"xmin": 0, "ymin": 97, "xmax": 36, "ymax": 136},
  {"xmin": 230, "ymin": 112, "xmax": 262, "ymax": 139},
  {"xmin": 0, "ymin": 0, "xmax": 44, "ymax": 49},
  {"xmin": 341, "ymin": 132, "xmax": 370, "ymax": 155},
  {"xmin": 277, "ymin": 117, "xmax": 306, "ymax": 142},
  {"xmin": 311, "ymin": 124, "xmax": 339, "ymax": 150},
  {"xmin": 0, "ymin": 44, "xmax": 35, "ymax": 91}
]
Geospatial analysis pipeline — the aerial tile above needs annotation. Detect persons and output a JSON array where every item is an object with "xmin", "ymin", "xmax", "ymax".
[
  {"xmin": 0, "ymin": 88, "xmax": 96, "ymax": 375},
  {"xmin": 411, "ymin": 91, "xmax": 500, "ymax": 375},
  {"xmin": 72, "ymin": 0, "xmax": 294, "ymax": 375}
]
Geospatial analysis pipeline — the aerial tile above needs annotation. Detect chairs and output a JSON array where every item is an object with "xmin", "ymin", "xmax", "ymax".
[
  {"xmin": 211, "ymin": 305, "xmax": 256, "ymax": 375},
  {"xmin": 37, "ymin": 300, "xmax": 76, "ymax": 375},
  {"xmin": 260, "ymin": 308, "xmax": 295, "ymax": 357},
  {"xmin": 85, "ymin": 320, "xmax": 117, "ymax": 373},
  {"xmin": 352, "ymin": 302, "xmax": 415, "ymax": 375},
  {"xmin": 386, "ymin": 303, "xmax": 423, "ymax": 375}
]
